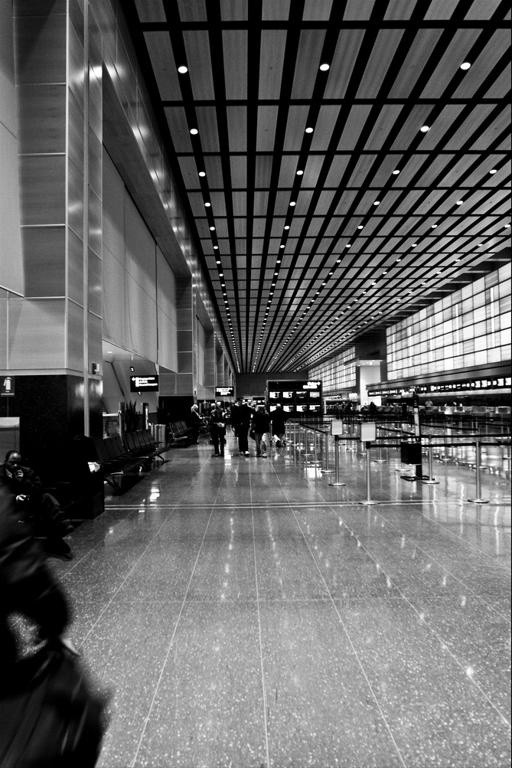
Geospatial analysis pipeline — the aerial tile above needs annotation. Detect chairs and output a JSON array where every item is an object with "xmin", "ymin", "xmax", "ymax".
[{"xmin": 102, "ymin": 419, "xmax": 198, "ymax": 499}]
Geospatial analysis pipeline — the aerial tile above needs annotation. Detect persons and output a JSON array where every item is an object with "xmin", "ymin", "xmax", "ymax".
[
  {"xmin": 0, "ymin": 480, "xmax": 72, "ymax": 706},
  {"xmin": 0, "ymin": 450, "xmax": 73, "ymax": 535},
  {"xmin": 326, "ymin": 399, "xmax": 434, "ymax": 416},
  {"xmin": 192, "ymin": 400, "xmax": 289, "ymax": 458}
]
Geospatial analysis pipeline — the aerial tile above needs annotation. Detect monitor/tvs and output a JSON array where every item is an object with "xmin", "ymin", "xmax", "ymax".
[
  {"xmin": 215, "ymin": 385, "xmax": 234, "ymax": 396},
  {"xmin": 130, "ymin": 374, "xmax": 159, "ymax": 392}
]
